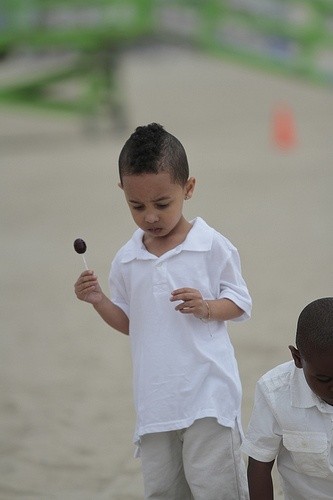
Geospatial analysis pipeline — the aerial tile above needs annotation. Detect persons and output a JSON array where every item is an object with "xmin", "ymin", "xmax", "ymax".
[
  {"xmin": 70, "ymin": 123, "xmax": 254, "ymax": 500},
  {"xmin": 244, "ymin": 296, "xmax": 333, "ymax": 499}
]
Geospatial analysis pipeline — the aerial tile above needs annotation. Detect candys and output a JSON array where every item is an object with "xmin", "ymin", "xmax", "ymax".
[{"xmin": 74, "ymin": 238, "xmax": 90, "ymax": 272}]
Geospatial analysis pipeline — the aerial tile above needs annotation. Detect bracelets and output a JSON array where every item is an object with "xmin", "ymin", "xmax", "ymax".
[{"xmin": 200, "ymin": 300, "xmax": 210, "ymax": 324}]
